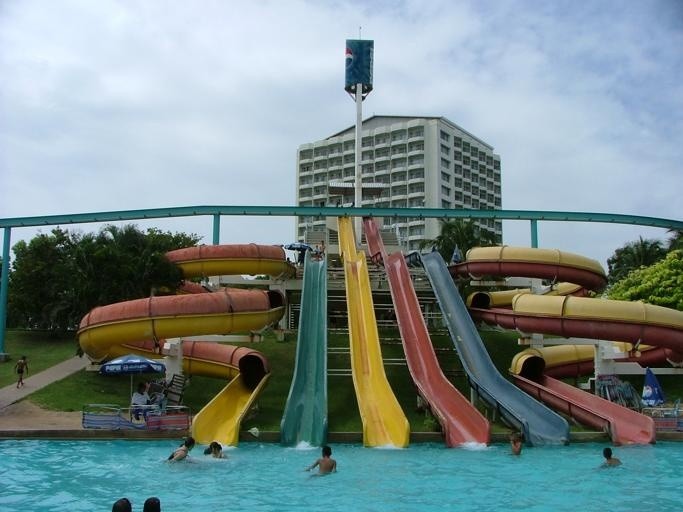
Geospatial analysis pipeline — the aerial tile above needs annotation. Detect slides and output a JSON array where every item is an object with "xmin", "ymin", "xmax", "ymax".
[
  {"xmin": 338, "ymin": 217, "xmax": 411, "ymax": 451},
  {"xmin": 466, "ymin": 247, "xmax": 682, "ymax": 444},
  {"xmin": 80, "ymin": 243, "xmax": 287, "ymax": 448},
  {"xmin": 280, "ymin": 247, "xmax": 328, "ymax": 450},
  {"xmin": 421, "ymin": 249, "xmax": 568, "ymax": 448},
  {"xmin": 363, "ymin": 219, "xmax": 490, "ymax": 448}
]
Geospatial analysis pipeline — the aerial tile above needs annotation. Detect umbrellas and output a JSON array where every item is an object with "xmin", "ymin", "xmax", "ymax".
[
  {"xmin": 641, "ymin": 367, "xmax": 666, "ymax": 408},
  {"xmin": 284, "ymin": 242, "xmax": 312, "ymax": 252},
  {"xmin": 451, "ymin": 244, "xmax": 463, "ymax": 265},
  {"xmin": 98, "ymin": 354, "xmax": 164, "ymax": 400}
]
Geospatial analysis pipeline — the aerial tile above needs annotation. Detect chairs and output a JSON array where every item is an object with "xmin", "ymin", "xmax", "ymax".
[
  {"xmin": 663, "ymin": 398, "xmax": 681, "ymax": 417},
  {"xmin": 127, "ymin": 373, "xmax": 187, "ymax": 424}
]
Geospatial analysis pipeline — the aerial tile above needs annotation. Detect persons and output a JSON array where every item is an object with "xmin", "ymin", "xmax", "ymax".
[
  {"xmin": 111, "ymin": 498, "xmax": 132, "ymax": 511},
  {"xmin": 142, "ymin": 496, "xmax": 161, "ymax": 512},
  {"xmin": 313, "ymin": 245, "xmax": 322, "ymax": 261},
  {"xmin": 594, "ymin": 447, "xmax": 622, "ymax": 471},
  {"xmin": 509, "ymin": 430, "xmax": 524, "ymax": 454},
  {"xmin": 318, "ymin": 240, "xmax": 325, "ymax": 254},
  {"xmin": 167, "ymin": 436, "xmax": 195, "ymax": 463},
  {"xmin": 286, "ymin": 257, "xmax": 292, "ymax": 264},
  {"xmin": 13, "ymin": 355, "xmax": 29, "ymax": 389},
  {"xmin": 304, "ymin": 445, "xmax": 336, "ymax": 477},
  {"xmin": 210, "ymin": 439, "xmax": 224, "ymax": 458},
  {"xmin": 130, "ymin": 382, "xmax": 150, "ymax": 422}
]
